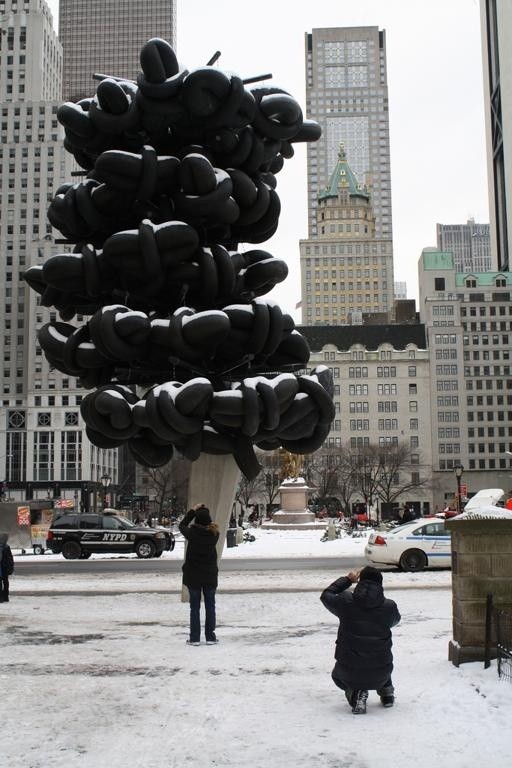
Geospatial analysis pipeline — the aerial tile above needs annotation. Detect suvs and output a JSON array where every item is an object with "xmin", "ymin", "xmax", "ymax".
[{"xmin": 46, "ymin": 513, "xmax": 176, "ymax": 560}]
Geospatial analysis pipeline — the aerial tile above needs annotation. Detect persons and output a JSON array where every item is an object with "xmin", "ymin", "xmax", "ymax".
[
  {"xmin": 179, "ymin": 502, "xmax": 220, "ymax": 646},
  {"xmin": 320, "ymin": 565, "xmax": 401, "ymax": 715},
  {"xmin": 335, "ymin": 509, "xmax": 357, "ymax": 530},
  {"xmin": 397, "ymin": 504, "xmax": 413, "ymax": 525},
  {"xmin": 0, "ymin": 531, "xmax": 14, "ymax": 603},
  {"xmin": 229, "ymin": 514, "xmax": 246, "ymax": 530}
]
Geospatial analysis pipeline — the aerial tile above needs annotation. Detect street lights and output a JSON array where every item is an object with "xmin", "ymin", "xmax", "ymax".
[
  {"xmin": 452, "ymin": 464, "xmax": 464, "ymax": 513},
  {"xmin": 45, "ymin": 486, "xmax": 51, "ymax": 497},
  {"xmin": 0, "ymin": 452, "xmax": 12, "ymax": 459},
  {"xmin": 504, "ymin": 451, "xmax": 512, "ymax": 459},
  {"xmin": 100, "ymin": 474, "xmax": 112, "ymax": 510}
]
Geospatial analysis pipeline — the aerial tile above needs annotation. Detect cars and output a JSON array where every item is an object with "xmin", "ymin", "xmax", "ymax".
[{"xmin": 363, "ymin": 516, "xmax": 453, "ymax": 573}]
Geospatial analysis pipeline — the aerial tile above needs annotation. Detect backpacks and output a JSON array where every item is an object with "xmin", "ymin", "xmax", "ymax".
[{"xmin": 2, "ymin": 546, "xmax": 14, "ymax": 574}]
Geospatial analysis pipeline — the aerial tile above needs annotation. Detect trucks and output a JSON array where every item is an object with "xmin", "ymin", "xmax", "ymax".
[{"xmin": 0, "ymin": 495, "xmax": 80, "ymax": 555}]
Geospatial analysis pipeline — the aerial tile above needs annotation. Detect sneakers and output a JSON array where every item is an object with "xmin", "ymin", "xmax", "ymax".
[
  {"xmin": 186, "ymin": 639, "xmax": 200, "ymax": 646},
  {"xmin": 207, "ymin": 638, "xmax": 219, "ymax": 644},
  {"xmin": 352, "ymin": 688, "xmax": 368, "ymax": 714},
  {"xmin": 380, "ymin": 696, "xmax": 393, "ymax": 706}
]
